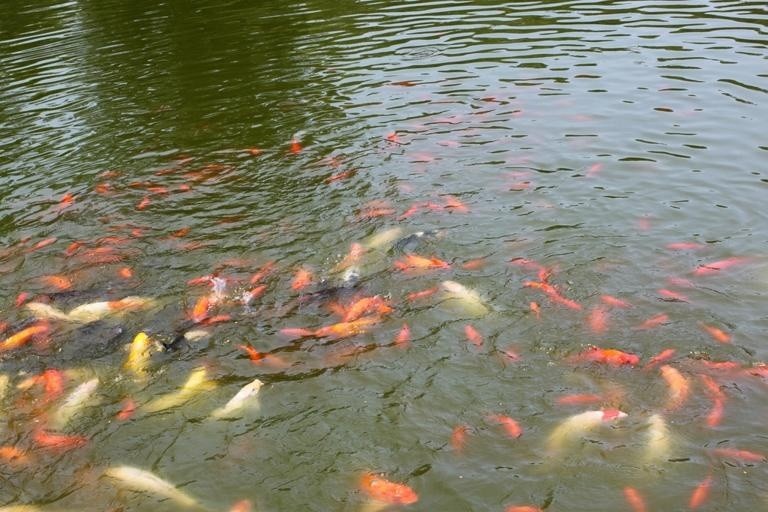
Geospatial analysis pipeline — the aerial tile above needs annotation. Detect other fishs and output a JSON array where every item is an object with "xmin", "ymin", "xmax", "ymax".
[{"xmin": 0, "ymin": 81, "xmax": 768, "ymax": 469}]
